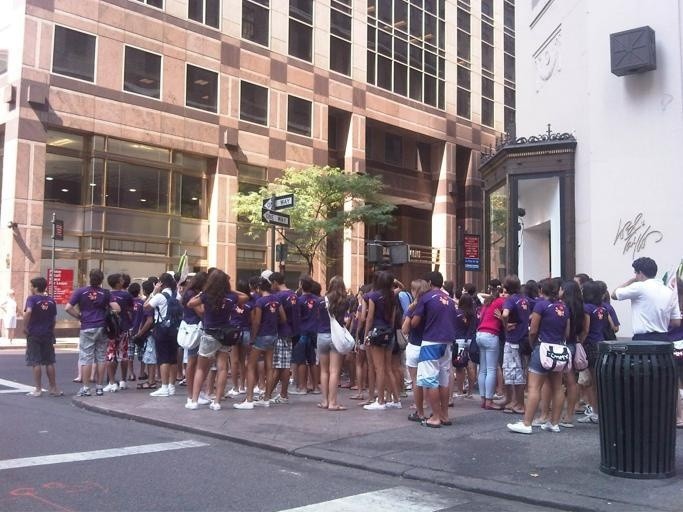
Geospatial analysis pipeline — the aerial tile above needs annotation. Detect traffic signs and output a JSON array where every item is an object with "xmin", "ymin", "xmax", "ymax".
[
  {"xmin": 261, "ymin": 209, "xmax": 289, "ymax": 227},
  {"xmin": 262, "ymin": 193, "xmax": 294, "ymax": 211}
]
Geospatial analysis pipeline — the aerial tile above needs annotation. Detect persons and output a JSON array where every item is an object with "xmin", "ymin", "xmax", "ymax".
[
  {"xmin": 0, "ymin": 289, "xmax": 25, "ymax": 342},
  {"xmin": 21, "ymin": 276, "xmax": 65, "ymax": 398}
]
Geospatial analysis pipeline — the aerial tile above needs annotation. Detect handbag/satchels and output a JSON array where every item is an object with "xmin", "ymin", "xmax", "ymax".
[
  {"xmin": 396, "ymin": 327, "xmax": 408, "ymax": 352},
  {"xmin": 452, "ymin": 343, "xmax": 468, "ymax": 369},
  {"xmin": 368, "ymin": 324, "xmax": 395, "ymax": 348},
  {"xmin": 329, "ymin": 318, "xmax": 356, "ymax": 356},
  {"xmin": 535, "ymin": 338, "xmax": 574, "ymax": 376},
  {"xmin": 573, "ymin": 341, "xmax": 588, "ymax": 372},
  {"xmin": 209, "ymin": 325, "xmax": 242, "ymax": 347},
  {"xmin": 468, "ymin": 334, "xmax": 489, "ymax": 367},
  {"xmin": 176, "ymin": 319, "xmax": 205, "ymax": 352}
]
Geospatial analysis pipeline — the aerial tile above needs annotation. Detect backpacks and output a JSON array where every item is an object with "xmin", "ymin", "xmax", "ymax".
[
  {"xmin": 159, "ymin": 291, "xmax": 182, "ymax": 328},
  {"xmin": 104, "ymin": 289, "xmax": 124, "ymax": 340}
]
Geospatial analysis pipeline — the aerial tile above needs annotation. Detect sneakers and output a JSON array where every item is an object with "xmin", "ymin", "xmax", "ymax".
[
  {"xmin": 315, "ymin": 382, "xmax": 522, "ymax": 427},
  {"xmin": 25, "ymin": 370, "xmax": 321, "ymax": 412},
  {"xmin": 507, "ymin": 401, "xmax": 600, "ymax": 434}
]
